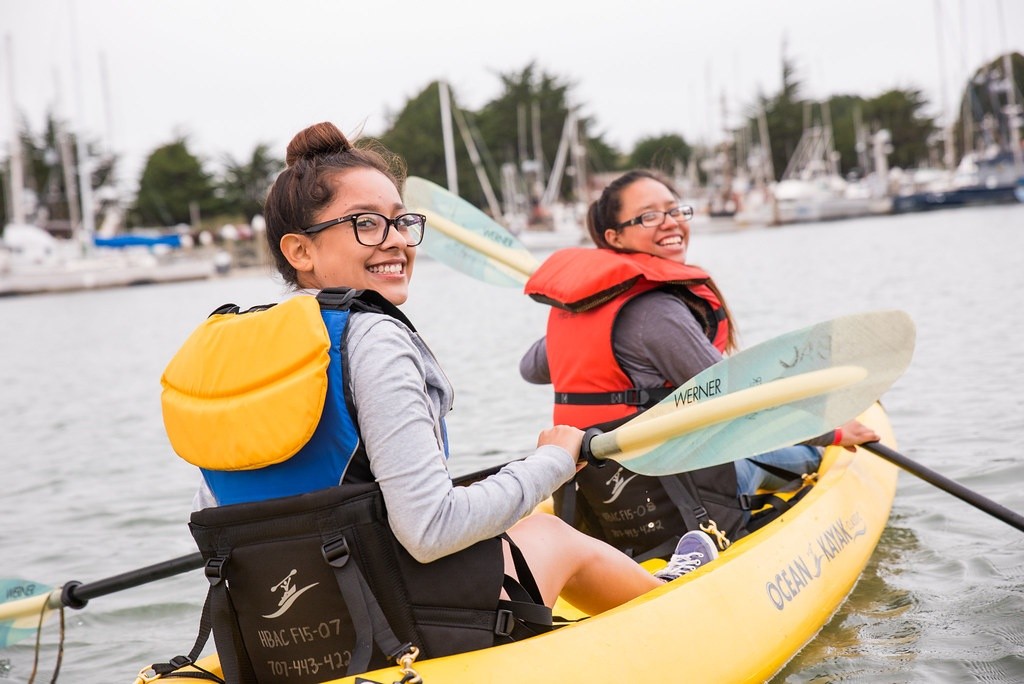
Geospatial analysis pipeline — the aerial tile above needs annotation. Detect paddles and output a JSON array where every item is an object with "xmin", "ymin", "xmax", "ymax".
[
  {"xmin": 0, "ymin": 309, "xmax": 918, "ymax": 662},
  {"xmin": 398, "ymin": 175, "xmax": 1024, "ymax": 535}
]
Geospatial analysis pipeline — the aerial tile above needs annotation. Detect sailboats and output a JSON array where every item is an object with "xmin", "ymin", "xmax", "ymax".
[{"xmin": 1, "ymin": 0, "xmax": 1024, "ymax": 299}]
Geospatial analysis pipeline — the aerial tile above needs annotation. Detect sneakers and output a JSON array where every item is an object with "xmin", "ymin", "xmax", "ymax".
[{"xmin": 653, "ymin": 530, "xmax": 718, "ymax": 580}]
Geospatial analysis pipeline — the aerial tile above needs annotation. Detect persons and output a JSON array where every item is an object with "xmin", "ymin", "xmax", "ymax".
[
  {"xmin": 160, "ymin": 122, "xmax": 717, "ymax": 616},
  {"xmin": 519, "ymin": 171, "xmax": 882, "ymax": 528}
]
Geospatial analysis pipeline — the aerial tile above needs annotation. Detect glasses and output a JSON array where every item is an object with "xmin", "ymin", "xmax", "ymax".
[
  {"xmin": 300, "ymin": 212, "xmax": 427, "ymax": 246},
  {"xmin": 614, "ymin": 205, "xmax": 694, "ymax": 228}
]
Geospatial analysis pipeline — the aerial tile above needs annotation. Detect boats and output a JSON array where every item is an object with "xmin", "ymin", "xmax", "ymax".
[{"xmin": 132, "ymin": 399, "xmax": 899, "ymax": 684}]
